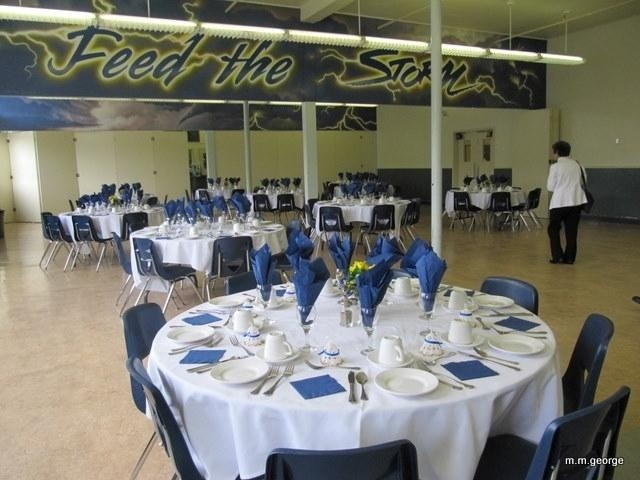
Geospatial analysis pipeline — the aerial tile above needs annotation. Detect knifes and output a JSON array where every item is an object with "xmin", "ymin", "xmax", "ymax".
[
  {"xmin": 458, "ymin": 351, "xmax": 521, "ymax": 372},
  {"xmin": 347, "ymin": 371, "xmax": 358, "ymax": 404}
]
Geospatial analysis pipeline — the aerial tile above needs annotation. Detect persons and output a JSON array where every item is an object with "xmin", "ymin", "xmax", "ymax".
[{"xmin": 545, "ymin": 140, "xmax": 587, "ymax": 264}]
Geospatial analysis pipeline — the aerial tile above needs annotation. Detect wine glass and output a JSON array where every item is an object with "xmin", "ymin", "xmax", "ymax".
[
  {"xmin": 358, "ymin": 305, "xmax": 377, "ymax": 356},
  {"xmin": 416, "ymin": 291, "xmax": 439, "ymax": 336},
  {"xmin": 296, "ymin": 304, "xmax": 318, "ymax": 353},
  {"xmin": 335, "ymin": 269, "xmax": 350, "ymax": 306},
  {"xmin": 256, "ymin": 283, "xmax": 275, "ymax": 326}
]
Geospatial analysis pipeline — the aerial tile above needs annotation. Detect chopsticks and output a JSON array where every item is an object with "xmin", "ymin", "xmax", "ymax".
[{"xmin": 492, "ymin": 309, "xmax": 534, "ymax": 316}]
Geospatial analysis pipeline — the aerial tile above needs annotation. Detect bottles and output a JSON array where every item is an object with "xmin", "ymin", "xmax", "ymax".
[{"xmin": 340, "ymin": 302, "xmax": 354, "ymax": 328}]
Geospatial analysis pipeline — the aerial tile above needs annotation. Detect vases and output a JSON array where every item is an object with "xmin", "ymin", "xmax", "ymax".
[{"xmin": 338, "ymin": 297, "xmax": 353, "ymax": 327}]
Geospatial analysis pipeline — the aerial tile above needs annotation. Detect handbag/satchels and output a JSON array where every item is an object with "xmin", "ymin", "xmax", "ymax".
[{"xmin": 581, "ymin": 189, "xmax": 596, "ymax": 215}]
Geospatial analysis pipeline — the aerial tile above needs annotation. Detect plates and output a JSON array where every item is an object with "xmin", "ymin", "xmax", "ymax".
[
  {"xmin": 209, "ymin": 358, "xmax": 268, "ymax": 385},
  {"xmin": 368, "ymin": 349, "xmax": 413, "ymax": 369},
  {"xmin": 440, "ymin": 330, "xmax": 486, "ymax": 347},
  {"xmin": 489, "ymin": 334, "xmax": 544, "ymax": 355},
  {"xmin": 225, "ymin": 322, "xmax": 263, "ymax": 337},
  {"xmin": 208, "ymin": 297, "xmax": 244, "ymax": 310},
  {"xmin": 442, "ymin": 301, "xmax": 479, "ymax": 313},
  {"xmin": 374, "ymin": 368, "xmax": 440, "ymax": 398},
  {"xmin": 257, "ymin": 346, "xmax": 300, "ymax": 364},
  {"xmin": 475, "ymin": 294, "xmax": 514, "ymax": 308},
  {"xmin": 165, "ymin": 325, "xmax": 215, "ymax": 345},
  {"xmin": 387, "ymin": 288, "xmax": 419, "ymax": 299}
]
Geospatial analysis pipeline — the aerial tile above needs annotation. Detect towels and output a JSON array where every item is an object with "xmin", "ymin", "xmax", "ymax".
[
  {"xmin": 498, "ymin": 174, "xmax": 509, "ymax": 183},
  {"xmin": 216, "ymin": 177, "xmax": 221, "ymax": 184},
  {"xmin": 213, "ymin": 195, "xmax": 228, "ymax": 216},
  {"xmin": 284, "ymin": 220, "xmax": 315, "ymax": 275},
  {"xmin": 464, "ymin": 176, "xmax": 473, "ymax": 185},
  {"xmin": 293, "ymin": 177, "xmax": 302, "ymax": 190},
  {"xmin": 356, "ymin": 259, "xmax": 392, "ymax": 329},
  {"xmin": 185, "ymin": 197, "xmax": 195, "ymax": 225},
  {"xmin": 327, "ymin": 233, "xmax": 352, "ymax": 285},
  {"xmin": 77, "ymin": 182, "xmax": 144, "ymax": 207},
  {"xmin": 274, "ymin": 179, "xmax": 280, "ymax": 190},
  {"xmin": 416, "ymin": 251, "xmax": 446, "ymax": 313},
  {"xmin": 196, "ymin": 200, "xmax": 214, "ymax": 223},
  {"xmin": 477, "ymin": 176, "xmax": 480, "ymax": 187},
  {"xmin": 261, "ymin": 177, "xmax": 268, "ymax": 190},
  {"xmin": 280, "ymin": 177, "xmax": 290, "ymax": 189},
  {"xmin": 175, "ymin": 198, "xmax": 185, "ymax": 218},
  {"xmin": 490, "ymin": 174, "xmax": 496, "ymax": 185},
  {"xmin": 229, "ymin": 192, "xmax": 251, "ymax": 215},
  {"xmin": 165, "ymin": 199, "xmax": 176, "ymax": 224},
  {"xmin": 229, "ymin": 177, "xmax": 235, "ymax": 185},
  {"xmin": 270, "ymin": 178, "xmax": 275, "ymax": 190},
  {"xmin": 235, "ymin": 177, "xmax": 240, "ymax": 184},
  {"xmin": 480, "ymin": 175, "xmax": 488, "ymax": 186},
  {"xmin": 208, "ymin": 178, "xmax": 214, "ymax": 185},
  {"xmin": 399, "ymin": 237, "xmax": 434, "ymax": 277},
  {"xmin": 288, "ymin": 258, "xmax": 331, "ymax": 325},
  {"xmin": 249, "ymin": 243, "xmax": 277, "ymax": 301},
  {"xmin": 366, "ymin": 234, "xmax": 404, "ymax": 269}
]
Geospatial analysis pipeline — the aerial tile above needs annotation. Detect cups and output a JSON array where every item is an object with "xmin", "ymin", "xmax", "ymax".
[
  {"xmin": 252, "ymin": 218, "xmax": 259, "ymax": 229},
  {"xmin": 379, "ymin": 198, "xmax": 385, "ymax": 205},
  {"xmin": 337, "ymin": 199, "xmax": 342, "ymax": 205},
  {"xmin": 361, "ymin": 199, "xmax": 365, "ymax": 206},
  {"xmin": 233, "ymin": 309, "xmax": 254, "ymax": 332},
  {"xmin": 379, "ymin": 335, "xmax": 406, "ymax": 364},
  {"xmin": 263, "ymin": 331, "xmax": 293, "ymax": 362},
  {"xmin": 233, "ymin": 223, "xmax": 241, "ymax": 234},
  {"xmin": 389, "ymin": 277, "xmax": 412, "ymax": 295},
  {"xmin": 163, "ymin": 222, "xmax": 170, "ymax": 231},
  {"xmin": 160, "ymin": 225, "xmax": 168, "ymax": 235},
  {"xmin": 189, "ymin": 226, "xmax": 198, "ymax": 236},
  {"xmin": 448, "ymin": 318, "xmax": 475, "ymax": 345},
  {"xmin": 321, "ymin": 277, "xmax": 333, "ymax": 294},
  {"xmin": 218, "ymin": 216, "xmax": 226, "ymax": 226},
  {"xmin": 448, "ymin": 289, "xmax": 474, "ymax": 310},
  {"xmin": 389, "ymin": 196, "xmax": 393, "ymax": 202},
  {"xmin": 333, "ymin": 197, "xmax": 338, "ymax": 202}
]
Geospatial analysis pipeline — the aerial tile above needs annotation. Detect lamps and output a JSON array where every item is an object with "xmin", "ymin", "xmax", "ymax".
[
  {"xmin": 362, "ymin": 29, "xmax": 429, "ymax": 53},
  {"xmin": 200, "ymin": 18, "xmax": 286, "ymax": 42},
  {"xmin": 485, "ymin": 1, "xmax": 539, "ymax": 62},
  {"xmin": 538, "ymin": 10, "xmax": 586, "ymax": 67},
  {"xmin": 287, "ymin": 1, "xmax": 363, "ymax": 48},
  {"xmin": 96, "ymin": 0, "xmax": 196, "ymax": 34},
  {"xmin": 0, "ymin": 1, "xmax": 95, "ymax": 26},
  {"xmin": 442, "ymin": 40, "xmax": 486, "ymax": 57}
]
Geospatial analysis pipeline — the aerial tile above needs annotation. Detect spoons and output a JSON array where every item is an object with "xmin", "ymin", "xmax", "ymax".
[
  {"xmin": 424, "ymin": 351, "xmax": 458, "ymax": 365},
  {"xmin": 476, "ymin": 316, "xmax": 490, "ymax": 331},
  {"xmin": 356, "ymin": 371, "xmax": 368, "ymax": 401},
  {"xmin": 474, "ymin": 346, "xmax": 519, "ymax": 367}
]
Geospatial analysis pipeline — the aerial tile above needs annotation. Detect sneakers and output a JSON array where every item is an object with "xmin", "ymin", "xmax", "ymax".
[
  {"xmin": 549, "ymin": 255, "xmax": 563, "ymax": 264},
  {"xmin": 561, "ymin": 257, "xmax": 575, "ymax": 264}
]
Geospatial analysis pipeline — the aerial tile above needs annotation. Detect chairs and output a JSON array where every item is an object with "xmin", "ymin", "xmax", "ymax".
[
  {"xmin": 270, "ymin": 251, "xmax": 295, "ymax": 282},
  {"xmin": 251, "ymin": 439, "xmax": 420, "ymax": 479},
  {"xmin": 307, "ymin": 198, "xmax": 320, "ymax": 237},
  {"xmin": 478, "ymin": 192, "xmax": 516, "ymax": 234},
  {"xmin": 109, "ymin": 212, "xmax": 148, "ymax": 266},
  {"xmin": 480, "ymin": 277, "xmax": 539, "ymax": 315},
  {"xmin": 450, "ymin": 192, "xmax": 486, "ymax": 233},
  {"xmin": 313, "ymin": 206, "xmax": 353, "ymax": 262},
  {"xmin": 70, "ymin": 214, "xmax": 119, "ymax": 273},
  {"xmin": 404, "ymin": 198, "xmax": 422, "ymax": 242},
  {"xmin": 273, "ymin": 194, "xmax": 296, "ymax": 224},
  {"xmin": 225, "ymin": 271, "xmax": 258, "ymax": 295},
  {"xmin": 471, "ymin": 385, "xmax": 630, "ymax": 479},
  {"xmin": 127, "ymin": 357, "xmax": 207, "ymax": 480},
  {"xmin": 201, "ymin": 235, "xmax": 253, "ymax": 302},
  {"xmin": 39, "ymin": 212, "xmax": 81, "ymax": 267},
  {"xmin": 69, "ymin": 200, "xmax": 76, "ymax": 211},
  {"xmin": 561, "ymin": 312, "xmax": 614, "ymax": 416},
  {"xmin": 252, "ymin": 194, "xmax": 277, "ymax": 223},
  {"xmin": 320, "ymin": 171, "xmax": 394, "ymax": 199},
  {"xmin": 147, "ymin": 197, "xmax": 160, "ymax": 206},
  {"xmin": 110, "ymin": 230, "xmax": 150, "ymax": 307},
  {"xmin": 123, "ymin": 302, "xmax": 168, "ymax": 480},
  {"xmin": 44, "ymin": 215, "xmax": 83, "ymax": 273},
  {"xmin": 225, "ymin": 189, "xmax": 245, "ymax": 219},
  {"xmin": 119, "ymin": 237, "xmax": 205, "ymax": 321},
  {"xmin": 502, "ymin": 188, "xmax": 542, "ymax": 233},
  {"xmin": 353, "ymin": 205, "xmax": 395, "ymax": 260}
]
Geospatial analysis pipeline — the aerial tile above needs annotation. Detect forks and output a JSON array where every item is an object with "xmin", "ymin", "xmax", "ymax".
[
  {"xmin": 436, "ymin": 379, "xmax": 463, "ymax": 390},
  {"xmin": 305, "ymin": 360, "xmax": 361, "ymax": 371},
  {"xmin": 189, "ymin": 311, "xmax": 233, "ymax": 319},
  {"xmin": 228, "ymin": 335, "xmax": 256, "ymax": 357},
  {"xmin": 172, "ymin": 333, "xmax": 220, "ymax": 352},
  {"xmin": 187, "ymin": 355, "xmax": 235, "ymax": 373},
  {"xmin": 482, "ymin": 312, "xmax": 527, "ymax": 318},
  {"xmin": 168, "ymin": 335, "xmax": 226, "ymax": 356},
  {"xmin": 264, "ymin": 360, "xmax": 297, "ymax": 396},
  {"xmin": 250, "ymin": 362, "xmax": 281, "ymax": 394},
  {"xmin": 493, "ymin": 326, "xmax": 548, "ymax": 335},
  {"xmin": 416, "ymin": 359, "xmax": 475, "ymax": 389},
  {"xmin": 196, "ymin": 308, "xmax": 234, "ymax": 314}
]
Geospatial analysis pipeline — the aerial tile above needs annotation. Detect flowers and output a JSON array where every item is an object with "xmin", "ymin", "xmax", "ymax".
[{"xmin": 336, "ymin": 262, "xmax": 372, "ymax": 306}]
